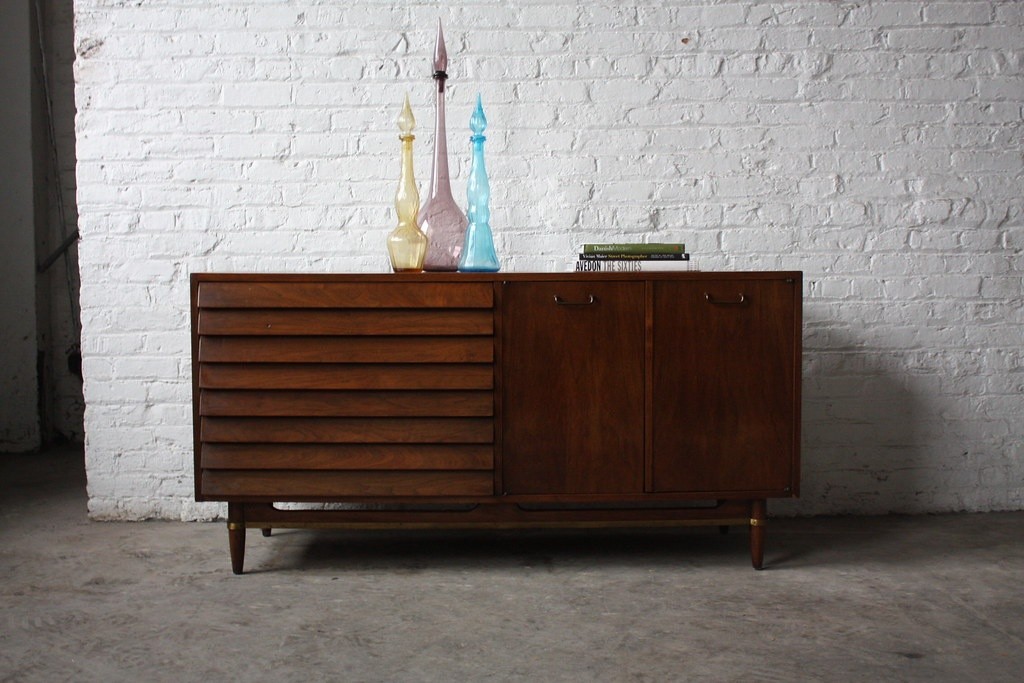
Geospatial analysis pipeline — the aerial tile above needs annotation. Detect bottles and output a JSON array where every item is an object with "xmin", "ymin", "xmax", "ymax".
[{"xmin": 388, "ymin": 15, "xmax": 501, "ymax": 273}]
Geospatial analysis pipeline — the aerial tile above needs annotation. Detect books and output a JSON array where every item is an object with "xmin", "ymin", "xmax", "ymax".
[{"xmin": 566, "ymin": 243, "xmax": 700, "ymax": 271}]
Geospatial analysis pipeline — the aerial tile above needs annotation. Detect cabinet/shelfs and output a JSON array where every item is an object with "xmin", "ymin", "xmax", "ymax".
[{"xmin": 190, "ymin": 270, "xmax": 804, "ymax": 574}]
